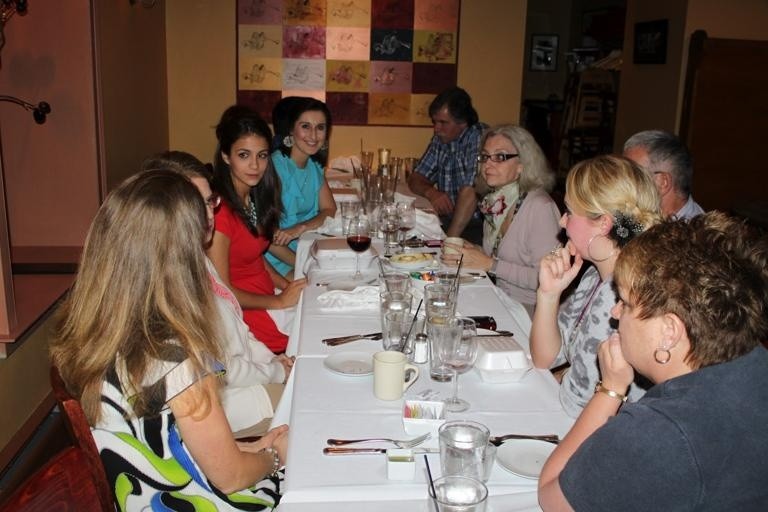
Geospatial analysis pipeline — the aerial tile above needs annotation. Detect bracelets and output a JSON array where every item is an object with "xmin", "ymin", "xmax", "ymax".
[
  {"xmin": 595, "ymin": 381, "xmax": 627, "ymax": 402},
  {"xmin": 257, "ymin": 447, "xmax": 280, "ymax": 478}
]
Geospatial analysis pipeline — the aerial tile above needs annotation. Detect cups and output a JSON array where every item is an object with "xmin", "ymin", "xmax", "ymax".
[
  {"xmin": 426, "ymin": 475, "xmax": 489, "ymax": 512},
  {"xmin": 438, "ymin": 419, "xmax": 490, "ymax": 485},
  {"xmin": 386, "ymin": 450, "xmax": 417, "ymax": 482},
  {"xmin": 340, "ymin": 149, "xmax": 405, "ymax": 202}
]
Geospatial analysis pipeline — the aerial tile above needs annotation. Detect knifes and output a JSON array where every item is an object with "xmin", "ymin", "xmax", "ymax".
[{"xmin": 323, "ymin": 447, "xmax": 442, "ymax": 455}]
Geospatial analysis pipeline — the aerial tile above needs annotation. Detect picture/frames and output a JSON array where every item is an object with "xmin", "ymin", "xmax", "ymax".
[
  {"xmin": 529, "ymin": 33, "xmax": 559, "ymax": 72},
  {"xmin": 633, "ymin": 19, "xmax": 667, "ymax": 64}
]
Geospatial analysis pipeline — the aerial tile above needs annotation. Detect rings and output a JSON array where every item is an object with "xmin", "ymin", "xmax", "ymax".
[{"xmin": 458, "ymin": 260, "xmax": 460, "ymax": 264}]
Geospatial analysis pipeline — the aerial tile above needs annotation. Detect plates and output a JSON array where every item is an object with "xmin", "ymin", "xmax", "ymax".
[
  {"xmin": 323, "ymin": 351, "xmax": 375, "ymax": 376},
  {"xmin": 328, "ymin": 281, "xmax": 369, "ymax": 292},
  {"xmin": 496, "ymin": 438, "xmax": 558, "ymax": 480}
]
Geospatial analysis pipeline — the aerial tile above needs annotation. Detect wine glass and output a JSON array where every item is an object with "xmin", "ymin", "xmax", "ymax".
[{"xmin": 347, "ymin": 201, "xmax": 479, "ymax": 412}]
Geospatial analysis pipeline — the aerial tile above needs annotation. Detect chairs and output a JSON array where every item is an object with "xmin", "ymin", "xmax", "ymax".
[{"xmin": 50, "ymin": 315, "xmax": 116, "ymax": 511}]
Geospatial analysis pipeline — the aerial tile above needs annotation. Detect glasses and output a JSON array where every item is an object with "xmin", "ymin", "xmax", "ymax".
[
  {"xmin": 205, "ymin": 196, "xmax": 223, "ymax": 209},
  {"xmin": 477, "ymin": 153, "xmax": 519, "ymax": 162}
]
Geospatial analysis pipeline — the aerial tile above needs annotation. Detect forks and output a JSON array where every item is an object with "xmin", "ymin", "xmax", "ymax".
[
  {"xmin": 326, "ymin": 432, "xmax": 432, "ymax": 449},
  {"xmin": 316, "ymin": 278, "xmax": 378, "ymax": 286}
]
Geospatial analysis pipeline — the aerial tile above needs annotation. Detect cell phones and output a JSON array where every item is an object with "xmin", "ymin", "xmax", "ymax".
[
  {"xmin": 457, "ymin": 316, "xmax": 497, "ymax": 331},
  {"xmin": 424, "ymin": 239, "xmax": 444, "ymax": 248}
]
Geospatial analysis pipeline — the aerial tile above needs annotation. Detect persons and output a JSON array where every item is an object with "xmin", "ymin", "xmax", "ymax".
[
  {"xmin": 139, "ymin": 150, "xmax": 297, "ymax": 439},
  {"xmin": 538, "ymin": 209, "xmax": 768, "ymax": 512},
  {"xmin": 529, "ymin": 153, "xmax": 665, "ymax": 419},
  {"xmin": 46, "ymin": 168, "xmax": 290, "ymax": 512},
  {"xmin": 441, "ymin": 125, "xmax": 562, "ymax": 322},
  {"xmin": 622, "ymin": 129, "xmax": 705, "ymax": 226},
  {"xmin": 408, "ymin": 88, "xmax": 495, "ymax": 247},
  {"xmin": 203, "ymin": 96, "xmax": 337, "ymax": 353}
]
